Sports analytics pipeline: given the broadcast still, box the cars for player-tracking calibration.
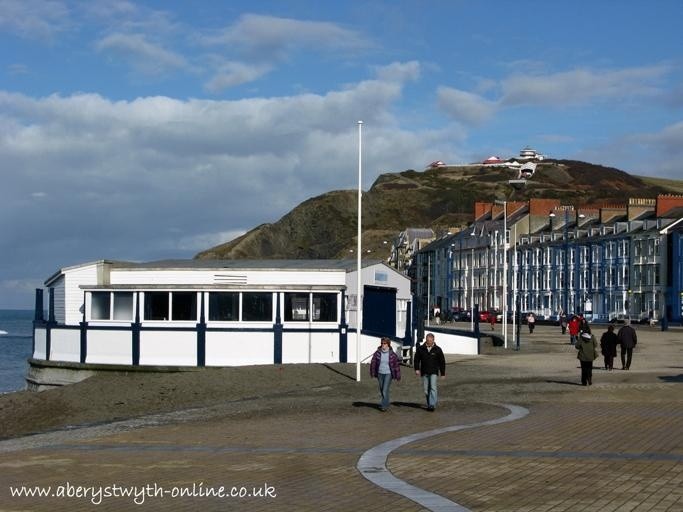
[438,309,537,324]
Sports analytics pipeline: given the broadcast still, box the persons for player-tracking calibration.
[423,309,457,327]
[414,333,446,412]
[490,314,496,330]
[370,336,401,412]
[527,313,536,334]
[649,308,656,328]
[559,310,588,346]
[574,326,598,386]
[600,326,618,371]
[617,319,637,370]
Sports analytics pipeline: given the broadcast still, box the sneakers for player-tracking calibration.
[605,367,629,371]
[582,379,592,386]
[424,404,435,411]
[379,405,389,411]
